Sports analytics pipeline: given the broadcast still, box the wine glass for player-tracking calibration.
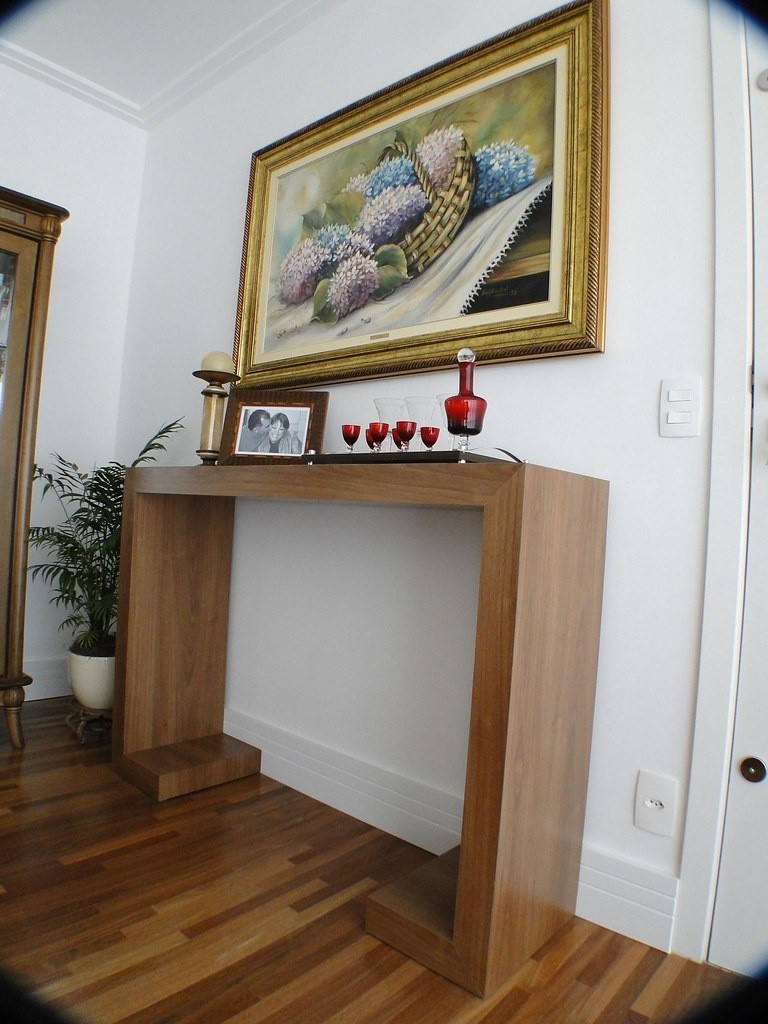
[341,421,440,453]
[373,397,405,452]
[404,396,443,451]
[436,393,458,451]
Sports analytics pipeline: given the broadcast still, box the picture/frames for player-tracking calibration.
[232,0,610,392]
[218,389,329,464]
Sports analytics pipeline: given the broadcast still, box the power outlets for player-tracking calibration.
[634,769,679,836]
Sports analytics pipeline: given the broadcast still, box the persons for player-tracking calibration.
[239,410,271,451]
[259,413,302,454]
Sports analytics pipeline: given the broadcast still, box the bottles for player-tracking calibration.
[444,347,487,451]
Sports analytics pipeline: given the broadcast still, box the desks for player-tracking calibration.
[107,461,609,999]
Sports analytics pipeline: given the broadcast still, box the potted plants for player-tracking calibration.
[26,419,185,745]
